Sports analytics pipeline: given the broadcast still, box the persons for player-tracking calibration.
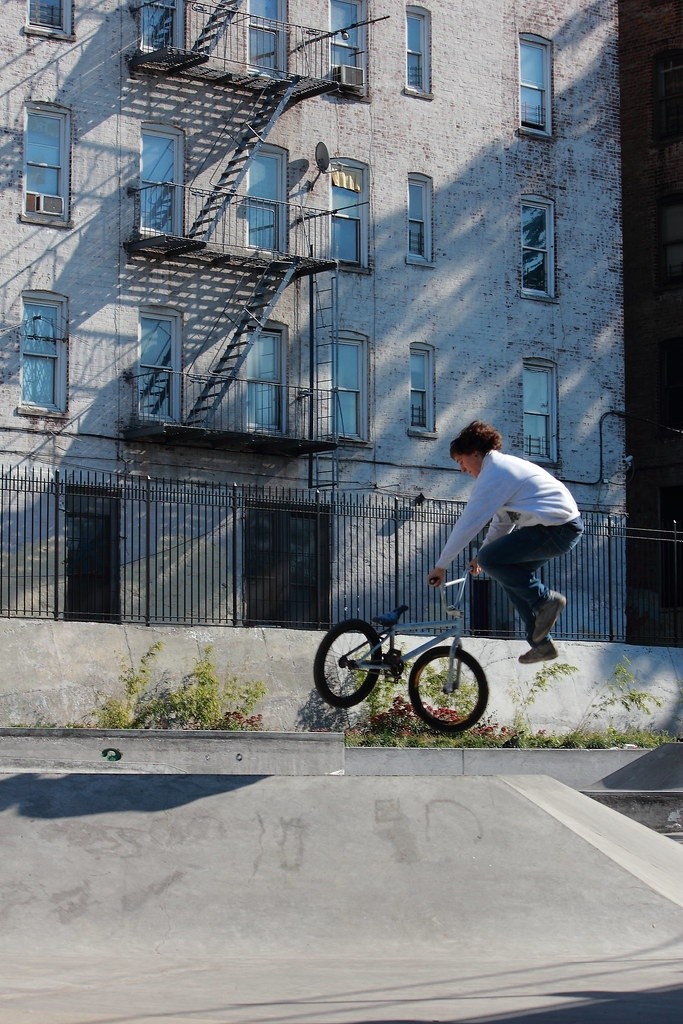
[424,420,584,662]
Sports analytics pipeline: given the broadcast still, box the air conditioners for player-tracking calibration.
[334,64,365,88]
[33,194,65,216]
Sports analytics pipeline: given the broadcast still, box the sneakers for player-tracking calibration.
[532,589,567,644]
[518,639,559,664]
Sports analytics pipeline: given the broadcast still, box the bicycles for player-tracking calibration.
[311,563,496,736]
